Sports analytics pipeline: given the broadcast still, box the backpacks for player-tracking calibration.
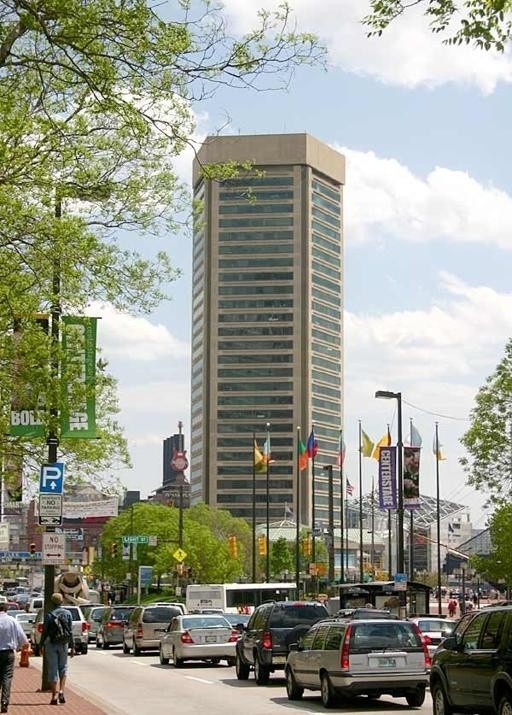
[49,610,73,645]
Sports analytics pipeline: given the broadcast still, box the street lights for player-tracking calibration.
[423,569,426,584]
[413,567,417,582]
[453,561,476,616]
[323,464,336,582]
[376,388,407,617]
[366,531,375,561]
[40,179,117,698]
[475,573,481,611]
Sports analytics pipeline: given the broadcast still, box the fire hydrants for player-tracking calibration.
[19,642,29,667]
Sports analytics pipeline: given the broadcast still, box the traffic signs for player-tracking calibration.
[41,532,65,566]
[121,536,148,544]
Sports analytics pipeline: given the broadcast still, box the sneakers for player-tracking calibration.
[50,699,57,705]
[59,692,65,703]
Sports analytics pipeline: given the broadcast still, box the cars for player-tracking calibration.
[405,617,458,673]
[0,574,44,636]
[158,613,239,669]
[221,613,251,632]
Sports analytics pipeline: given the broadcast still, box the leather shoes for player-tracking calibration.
[2,702,7,713]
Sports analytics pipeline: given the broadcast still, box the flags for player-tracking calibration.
[298,439,306,472]
[263,437,273,466]
[306,430,318,459]
[411,425,423,449]
[337,441,345,468]
[254,440,266,474]
[374,433,391,463]
[346,477,354,496]
[286,504,292,514]
[433,435,447,460]
[359,429,373,458]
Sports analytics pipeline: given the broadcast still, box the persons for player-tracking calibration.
[1,603,30,713]
[426,586,503,618]
[38,593,75,705]
[109,590,124,603]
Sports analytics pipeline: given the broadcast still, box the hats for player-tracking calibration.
[51,592,64,605]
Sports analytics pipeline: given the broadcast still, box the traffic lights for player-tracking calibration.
[259,536,266,558]
[227,536,237,560]
[302,537,310,558]
[110,542,117,560]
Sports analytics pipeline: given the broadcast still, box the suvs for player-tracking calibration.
[284,608,433,709]
[234,600,330,685]
[430,604,512,715]
[27,601,187,660]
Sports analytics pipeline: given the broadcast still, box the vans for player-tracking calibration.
[188,608,224,616]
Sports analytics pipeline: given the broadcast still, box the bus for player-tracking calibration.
[185,582,298,617]
[287,571,375,599]
